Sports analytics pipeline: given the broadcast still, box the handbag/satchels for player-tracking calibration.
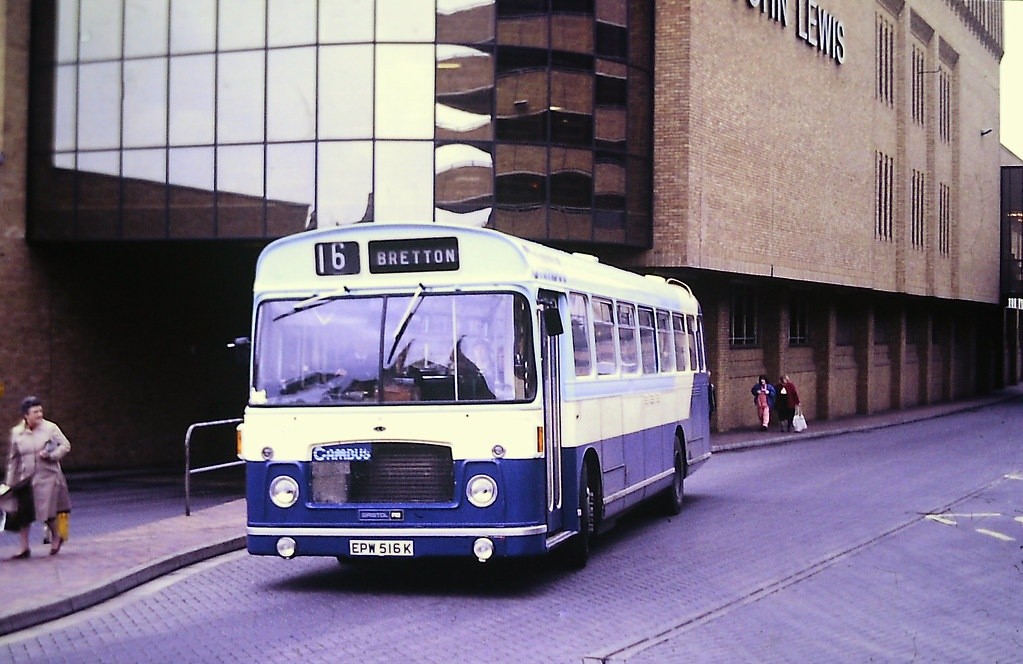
[793,404,808,433]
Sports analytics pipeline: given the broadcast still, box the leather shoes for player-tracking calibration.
[49,537,63,556]
[9,548,30,559]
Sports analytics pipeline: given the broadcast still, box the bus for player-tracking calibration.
[237,222,709,574]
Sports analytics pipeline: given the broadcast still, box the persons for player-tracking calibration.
[751,375,777,431]
[386,343,524,399]
[706,371,717,421]
[4,395,71,560]
[775,374,802,434]
